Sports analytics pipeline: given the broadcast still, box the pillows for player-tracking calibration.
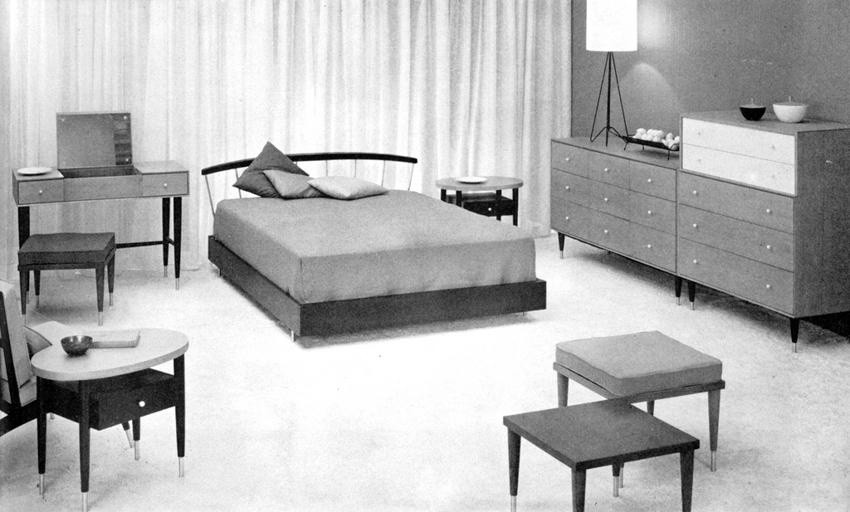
[233,141,309,199]
[23,325,53,356]
[262,170,324,199]
[307,175,389,199]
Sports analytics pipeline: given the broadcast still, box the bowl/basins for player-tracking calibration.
[61,335,92,356]
[772,101,809,124]
[740,105,766,120]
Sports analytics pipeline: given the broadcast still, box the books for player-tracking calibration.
[88,329,141,348]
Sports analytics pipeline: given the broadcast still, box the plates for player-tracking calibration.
[457,177,487,184]
[18,166,52,175]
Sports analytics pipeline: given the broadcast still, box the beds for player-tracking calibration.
[200,153,547,347]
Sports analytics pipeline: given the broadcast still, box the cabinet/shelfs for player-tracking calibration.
[12,112,190,279]
[551,137,681,304]
[677,104,848,344]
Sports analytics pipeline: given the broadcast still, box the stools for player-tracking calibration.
[503,398,700,512]
[15,233,116,327]
[553,331,726,487]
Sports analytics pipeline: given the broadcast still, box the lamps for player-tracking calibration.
[586,0,638,146]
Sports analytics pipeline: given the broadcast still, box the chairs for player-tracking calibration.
[0,280,82,438]
